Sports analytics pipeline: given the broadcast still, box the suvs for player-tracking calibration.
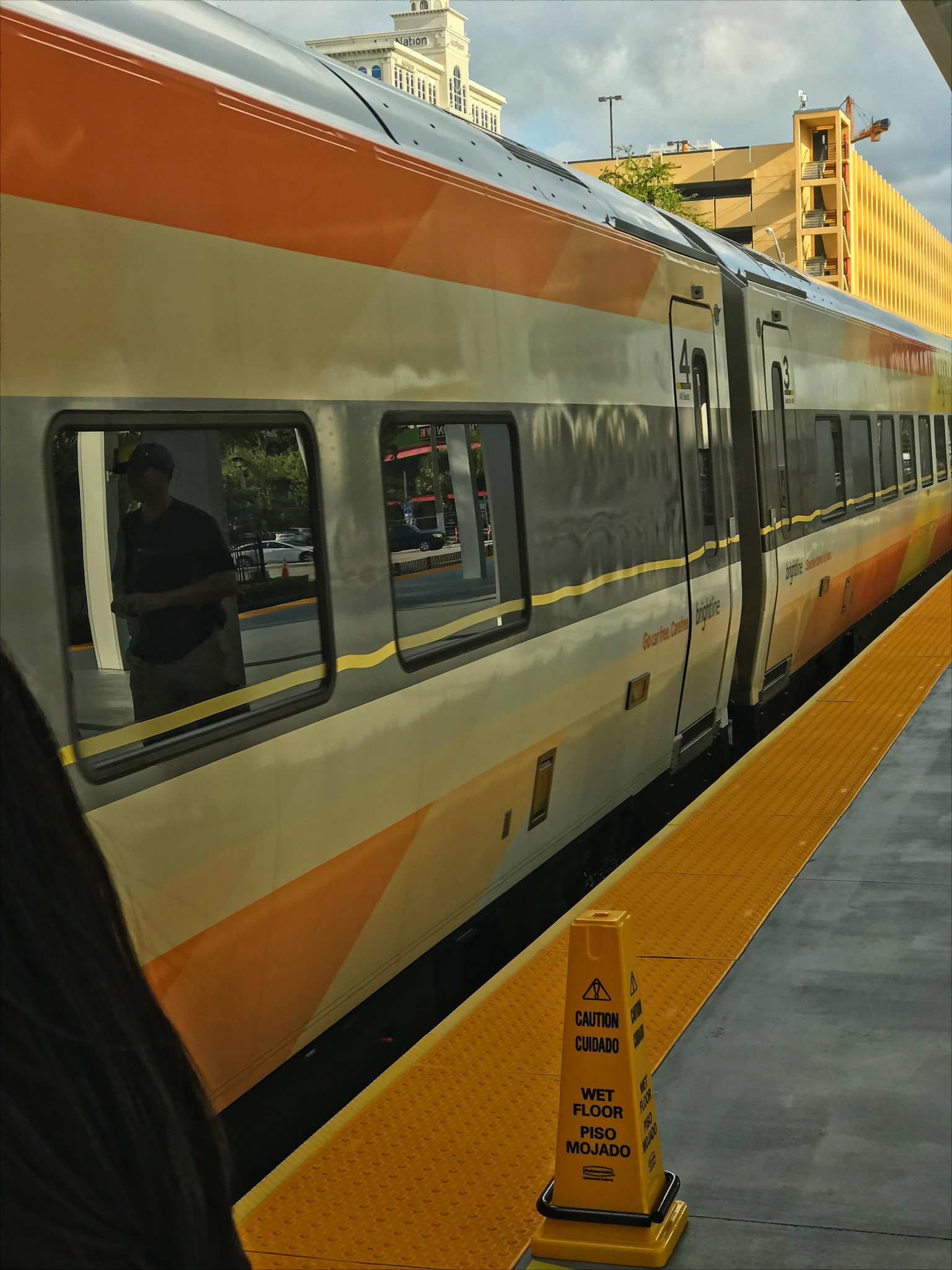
[388,524,444,552]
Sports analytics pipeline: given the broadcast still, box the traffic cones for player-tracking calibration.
[281,558,289,577]
[532,908,688,1268]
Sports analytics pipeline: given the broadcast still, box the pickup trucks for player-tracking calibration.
[275,527,313,546]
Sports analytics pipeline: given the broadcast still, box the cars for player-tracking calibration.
[230,541,313,567]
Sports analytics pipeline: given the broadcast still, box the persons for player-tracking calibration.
[109,442,247,748]
[0,646,247,1270]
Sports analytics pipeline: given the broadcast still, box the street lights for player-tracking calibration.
[598,95,623,157]
[667,141,689,152]
[765,227,784,263]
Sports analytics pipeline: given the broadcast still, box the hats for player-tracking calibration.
[114,441,174,474]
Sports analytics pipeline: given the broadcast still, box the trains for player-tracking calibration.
[1,2,951,1116]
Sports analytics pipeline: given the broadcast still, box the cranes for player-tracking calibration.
[845,96,892,149]
